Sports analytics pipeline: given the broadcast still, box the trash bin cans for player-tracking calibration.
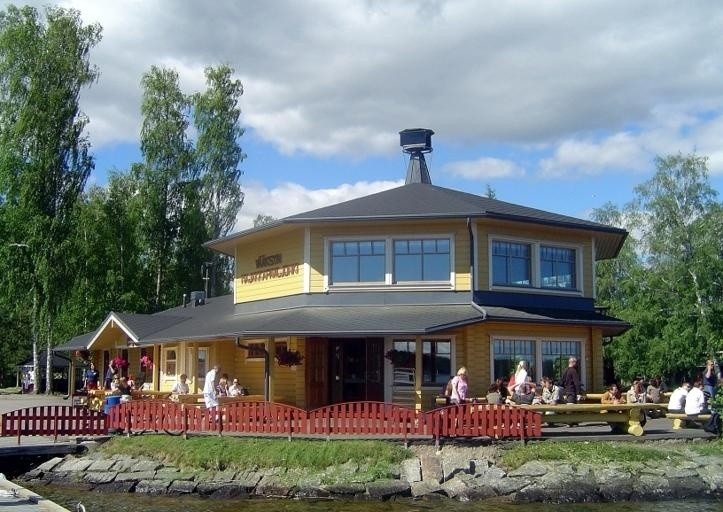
[104,396,121,413]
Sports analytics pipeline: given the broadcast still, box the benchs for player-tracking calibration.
[89,389,265,412]
[436,392,713,436]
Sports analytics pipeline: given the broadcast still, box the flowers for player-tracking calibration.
[275,350,303,368]
[140,355,153,370]
[113,355,128,368]
[386,350,415,366]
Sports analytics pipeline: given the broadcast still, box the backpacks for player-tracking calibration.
[703,413,721,434]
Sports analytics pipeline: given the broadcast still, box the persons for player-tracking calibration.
[442,354,717,428]
[80,359,245,423]
[27,367,34,383]
